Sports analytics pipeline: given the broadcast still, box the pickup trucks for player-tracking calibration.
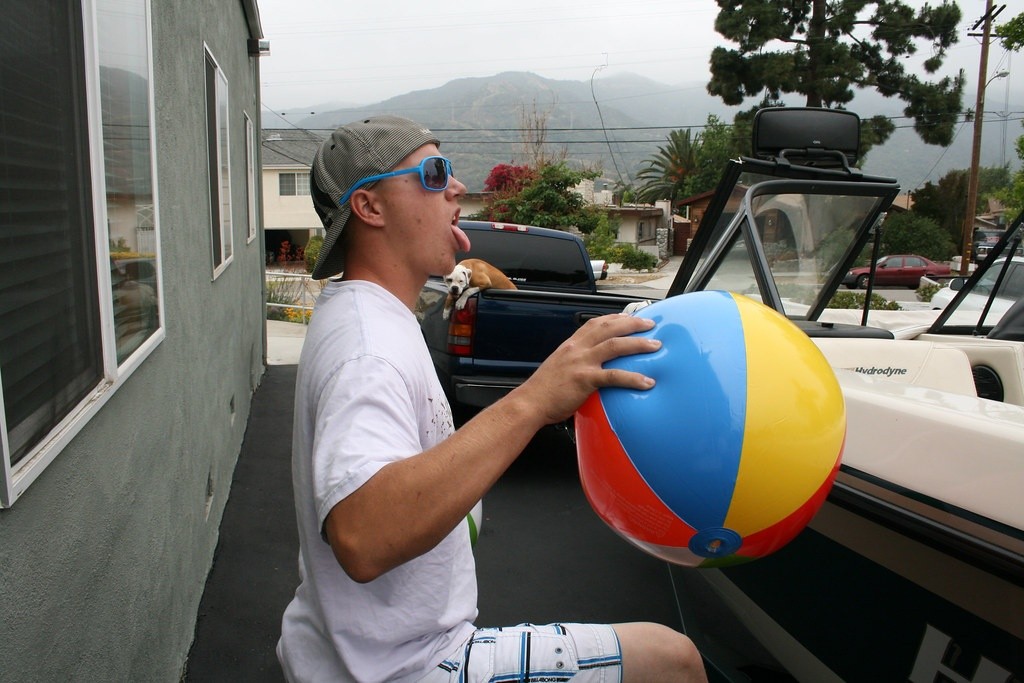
[111,260,158,305]
[415,222,667,450]
[590,259,607,280]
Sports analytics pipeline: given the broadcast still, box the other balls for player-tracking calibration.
[575,290,847,569]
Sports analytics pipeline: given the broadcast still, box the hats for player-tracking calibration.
[310,115,441,281]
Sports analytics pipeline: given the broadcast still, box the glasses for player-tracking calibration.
[340,156,454,205]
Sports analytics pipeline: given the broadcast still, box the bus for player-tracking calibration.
[975,228,1007,244]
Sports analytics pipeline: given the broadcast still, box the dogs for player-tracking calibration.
[442,258,518,320]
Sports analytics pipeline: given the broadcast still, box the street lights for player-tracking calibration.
[960,71,1011,282]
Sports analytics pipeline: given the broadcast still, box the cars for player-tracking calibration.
[840,254,952,288]
[931,257,1024,311]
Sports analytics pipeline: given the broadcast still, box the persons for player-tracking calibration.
[276,115,708,683]
